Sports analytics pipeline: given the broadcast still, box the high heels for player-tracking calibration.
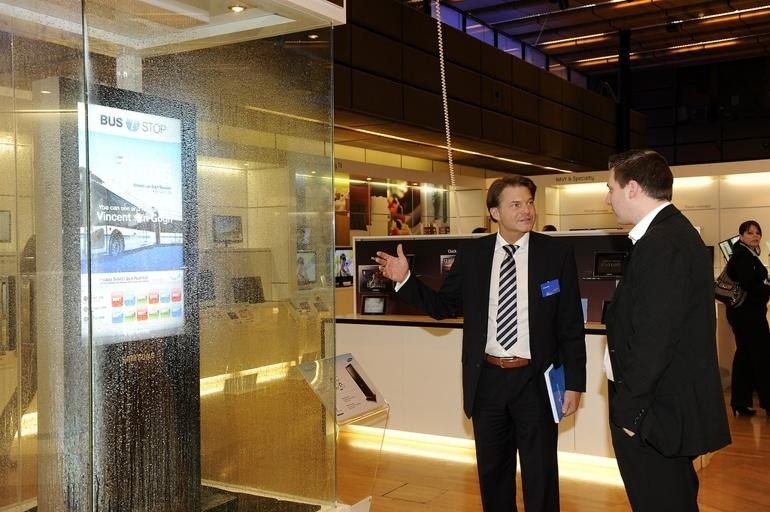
[731,403,756,416]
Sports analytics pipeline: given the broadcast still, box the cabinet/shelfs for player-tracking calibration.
[244,163,450,314]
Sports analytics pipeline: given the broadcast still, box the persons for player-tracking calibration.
[603,149,731,511]
[336,254,351,277]
[375,174,586,512]
[472,227,488,233]
[541,225,557,231]
[725,220,770,421]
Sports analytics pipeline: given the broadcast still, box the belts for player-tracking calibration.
[484,355,531,370]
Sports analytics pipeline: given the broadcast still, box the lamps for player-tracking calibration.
[228,4,247,14]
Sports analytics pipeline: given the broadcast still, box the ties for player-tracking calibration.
[496,245,519,351]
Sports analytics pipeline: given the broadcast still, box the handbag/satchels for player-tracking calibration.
[713,269,747,309]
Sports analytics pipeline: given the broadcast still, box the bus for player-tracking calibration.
[78,168,158,259]
[156,218,184,246]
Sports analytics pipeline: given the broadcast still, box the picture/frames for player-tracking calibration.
[0,276,16,351]
[718,234,761,264]
[0,210,11,243]
[297,251,317,284]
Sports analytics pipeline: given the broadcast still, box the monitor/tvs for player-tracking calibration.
[334,246,353,287]
[296,228,312,250]
[231,276,265,303]
[296,250,317,289]
[592,251,630,280]
[211,215,243,246]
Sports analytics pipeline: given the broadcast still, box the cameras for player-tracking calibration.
[358,265,393,295]
